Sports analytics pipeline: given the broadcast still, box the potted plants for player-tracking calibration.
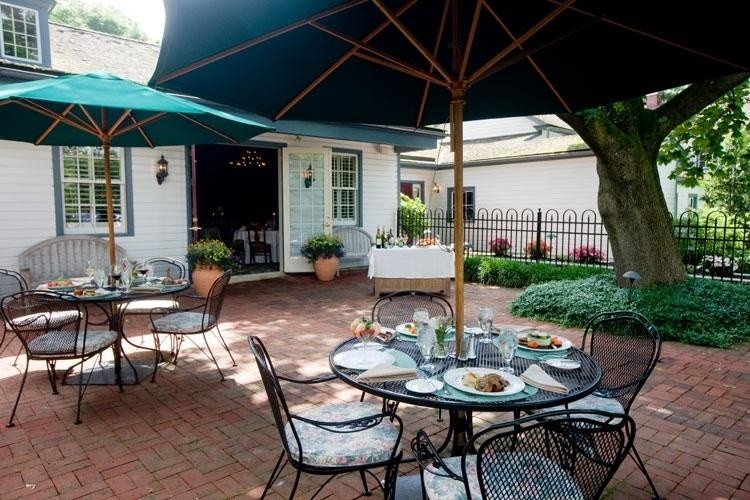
[301,234,344,281]
[185,238,234,295]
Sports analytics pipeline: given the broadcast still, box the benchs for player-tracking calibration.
[331,226,373,277]
[18,234,129,290]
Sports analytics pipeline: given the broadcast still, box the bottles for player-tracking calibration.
[458,333,477,361]
[376,224,393,248]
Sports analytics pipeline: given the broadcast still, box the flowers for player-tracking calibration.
[487,237,511,255]
[569,243,603,260]
[349,317,381,342]
[524,238,551,255]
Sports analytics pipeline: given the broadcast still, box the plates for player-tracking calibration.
[332,349,395,370]
[444,366,525,395]
[547,358,582,371]
[352,342,382,350]
[67,276,96,284]
[396,322,418,336]
[405,378,444,394]
[516,332,571,351]
[66,291,111,298]
[153,281,188,289]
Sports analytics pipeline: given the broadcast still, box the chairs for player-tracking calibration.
[409,408,636,500]
[371,290,454,422]
[247,334,403,500]
[518,311,664,499]
[247,223,272,267]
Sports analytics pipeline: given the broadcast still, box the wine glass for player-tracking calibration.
[498,329,518,374]
[418,327,437,371]
[94,270,105,289]
[479,305,493,344]
[413,309,429,346]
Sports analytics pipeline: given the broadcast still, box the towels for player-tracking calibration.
[520,363,569,395]
[354,362,417,384]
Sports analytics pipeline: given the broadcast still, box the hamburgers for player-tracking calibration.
[85,289,96,296]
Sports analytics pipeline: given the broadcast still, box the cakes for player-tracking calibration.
[526,330,552,346]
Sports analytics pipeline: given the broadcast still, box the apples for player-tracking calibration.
[74,288,83,296]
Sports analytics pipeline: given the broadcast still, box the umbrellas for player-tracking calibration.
[147,0,750,420]
[0,73,276,314]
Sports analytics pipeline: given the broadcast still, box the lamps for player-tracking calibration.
[156,153,168,185]
[304,163,316,187]
[433,181,440,194]
